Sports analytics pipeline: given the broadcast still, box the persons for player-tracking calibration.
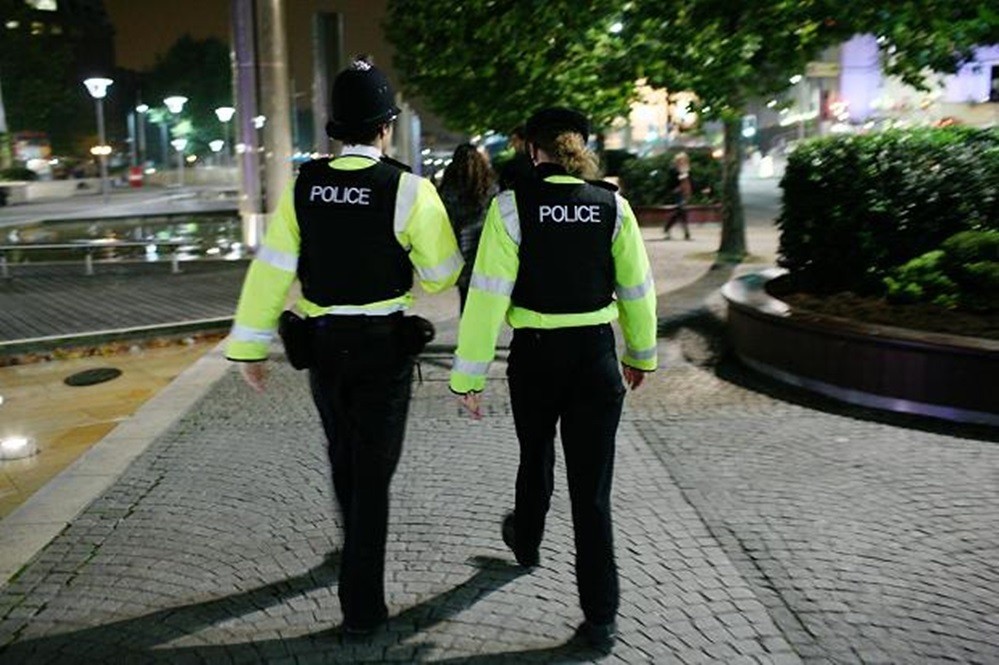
[661,151,711,243]
[437,143,498,316]
[449,100,658,652]
[491,124,537,190]
[219,52,466,639]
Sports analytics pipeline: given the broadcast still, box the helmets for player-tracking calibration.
[332,58,401,126]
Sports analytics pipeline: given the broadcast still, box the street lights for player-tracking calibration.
[210,105,265,175]
[85,77,114,193]
[162,95,189,192]
[134,103,150,163]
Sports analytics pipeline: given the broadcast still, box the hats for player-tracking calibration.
[527,107,589,143]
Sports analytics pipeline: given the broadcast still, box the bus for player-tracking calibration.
[1,129,50,170]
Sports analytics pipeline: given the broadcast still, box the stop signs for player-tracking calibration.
[127,165,145,187]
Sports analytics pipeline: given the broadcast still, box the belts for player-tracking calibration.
[314,319,399,329]
[516,327,611,336]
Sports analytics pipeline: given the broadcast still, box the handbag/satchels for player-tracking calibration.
[277,313,310,370]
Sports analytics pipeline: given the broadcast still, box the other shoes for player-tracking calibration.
[578,620,617,648]
[504,514,540,566]
[345,606,389,640]
